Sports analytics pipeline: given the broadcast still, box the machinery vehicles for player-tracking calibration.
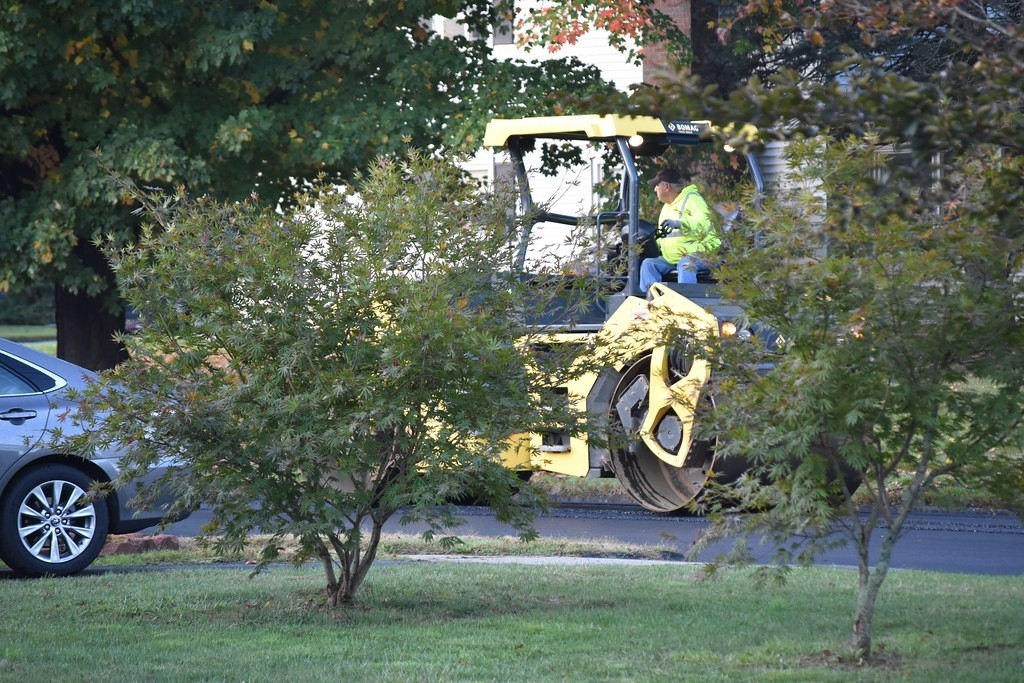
[299,113,875,515]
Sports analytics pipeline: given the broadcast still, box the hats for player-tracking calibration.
[646,167,684,186]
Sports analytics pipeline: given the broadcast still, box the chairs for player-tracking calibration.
[665,201,739,281]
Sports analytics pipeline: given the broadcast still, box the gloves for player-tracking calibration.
[660,218,682,235]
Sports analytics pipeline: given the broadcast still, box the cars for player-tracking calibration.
[0,335,212,580]
[124,279,205,337]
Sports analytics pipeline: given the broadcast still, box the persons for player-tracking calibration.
[640,167,726,293]
